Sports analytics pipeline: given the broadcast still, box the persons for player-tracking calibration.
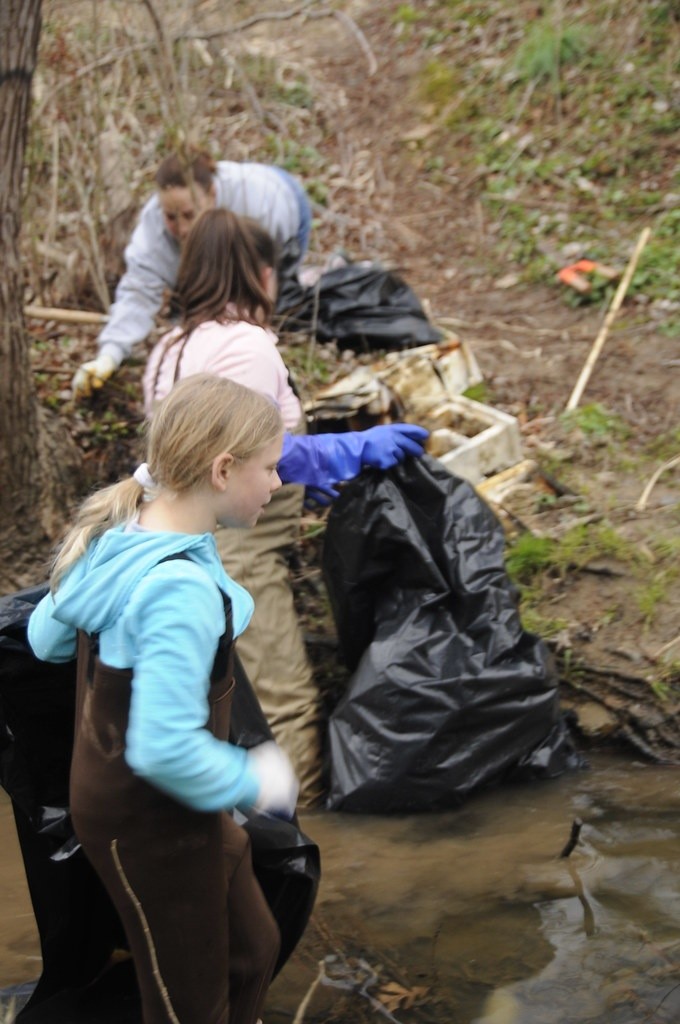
[143,208,429,814]
[27,370,301,1024]
[74,139,311,407]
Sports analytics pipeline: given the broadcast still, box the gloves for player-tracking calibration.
[69,352,127,408]
[247,740,300,821]
[276,423,429,509]
